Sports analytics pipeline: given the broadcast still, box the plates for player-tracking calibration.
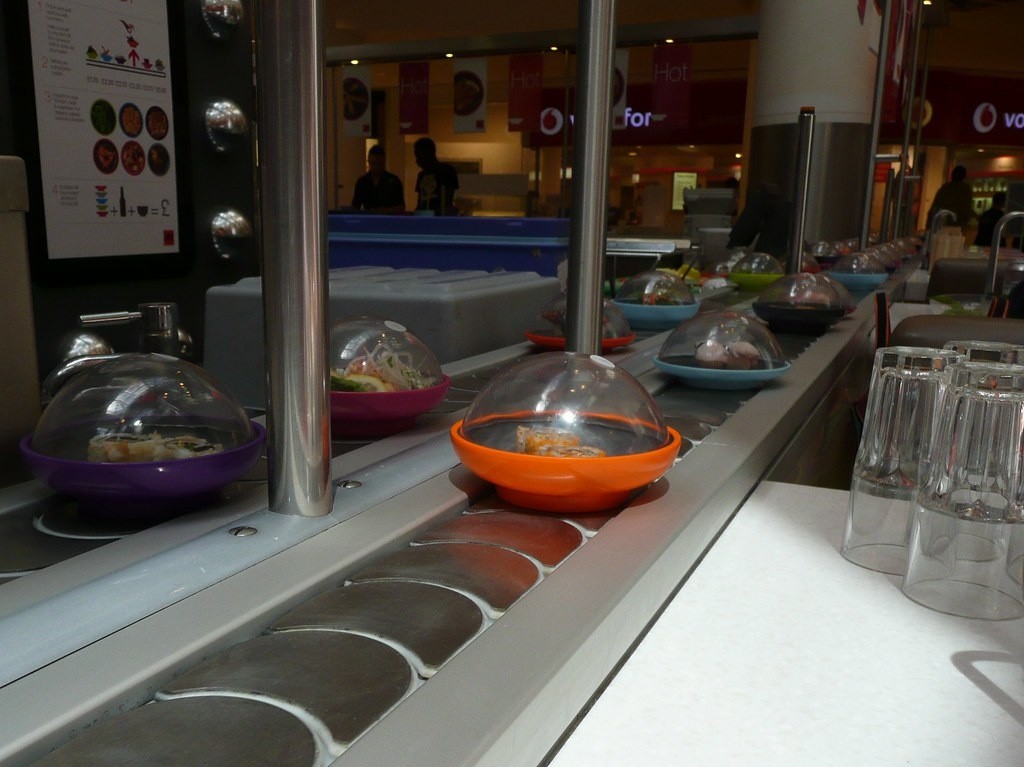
[119,103,143,138]
[92,138,119,175]
[89,99,117,136]
[120,140,145,176]
[344,77,368,121]
[145,105,169,141]
[613,66,624,109]
[454,70,483,115]
[147,143,170,177]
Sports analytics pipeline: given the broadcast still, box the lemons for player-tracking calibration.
[343,375,387,392]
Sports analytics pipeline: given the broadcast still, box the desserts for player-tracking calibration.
[693,339,760,369]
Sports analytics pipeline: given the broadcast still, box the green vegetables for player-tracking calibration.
[328,375,366,392]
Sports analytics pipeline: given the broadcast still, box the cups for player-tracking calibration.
[843,341,1023,621]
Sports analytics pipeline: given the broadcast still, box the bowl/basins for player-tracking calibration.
[813,255,844,265]
[19,413,267,506]
[330,374,450,436]
[752,301,845,332]
[822,269,888,290]
[930,293,995,318]
[652,353,792,391]
[609,298,701,329]
[841,241,922,274]
[524,327,636,354]
[451,413,681,514]
[728,272,785,289]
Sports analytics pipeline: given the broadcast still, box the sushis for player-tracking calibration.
[87,432,225,463]
[516,424,607,458]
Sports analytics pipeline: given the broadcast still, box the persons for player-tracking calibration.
[974,192,1008,246]
[727,162,790,252]
[413,136,459,217]
[350,144,406,213]
[924,164,972,231]
